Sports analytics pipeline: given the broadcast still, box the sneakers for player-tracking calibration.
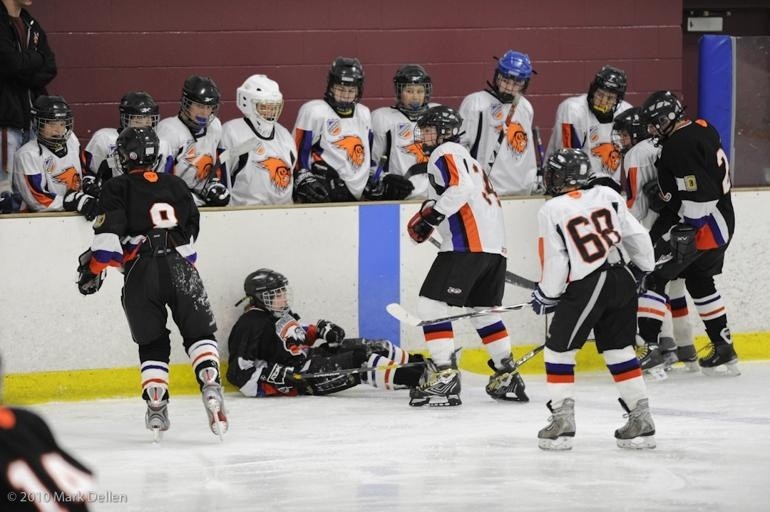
[410,346,461,397]
[195,360,229,435]
[486,352,524,394]
[634,329,737,376]
[143,387,170,432]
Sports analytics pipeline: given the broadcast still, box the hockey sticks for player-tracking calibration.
[387,303,533,326]
[208,138,260,183]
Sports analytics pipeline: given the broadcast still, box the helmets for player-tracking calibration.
[586,65,628,117]
[544,148,591,196]
[413,105,462,155]
[110,91,163,173]
[611,90,689,157]
[493,49,532,102]
[30,95,74,149]
[393,63,432,117]
[325,55,365,111]
[180,74,221,129]
[244,268,293,318]
[236,73,284,138]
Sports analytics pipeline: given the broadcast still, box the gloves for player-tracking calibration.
[644,180,665,211]
[382,174,414,200]
[670,227,697,263]
[407,198,445,244]
[531,282,561,315]
[205,185,230,207]
[78,247,107,295]
[294,171,329,203]
[63,176,103,221]
[317,318,345,343]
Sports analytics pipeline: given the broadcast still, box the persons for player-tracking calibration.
[82,90,175,191]
[216,72,299,208]
[0,0,58,215]
[226,268,437,397]
[633,90,737,371]
[454,49,548,198]
[406,104,527,399]
[76,124,230,437]
[0,405,89,511]
[540,65,636,200]
[288,55,374,205]
[612,106,699,367]
[12,93,102,221]
[526,146,656,440]
[364,63,445,203]
[155,72,230,208]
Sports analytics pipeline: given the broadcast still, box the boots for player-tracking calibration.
[616,398,655,439]
[538,399,575,439]
